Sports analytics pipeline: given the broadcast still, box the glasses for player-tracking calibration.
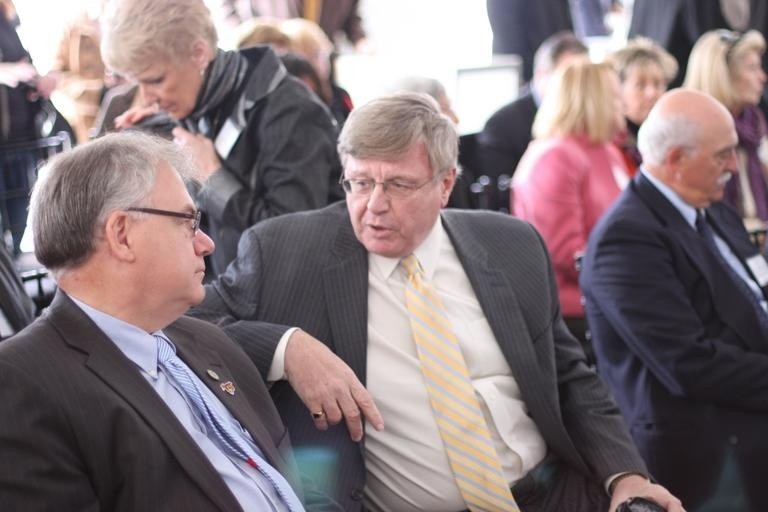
[339,162,442,197]
[125,207,203,234]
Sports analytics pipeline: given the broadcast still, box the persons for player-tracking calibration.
[98,1,342,299]
[231,23,320,114]
[0,0,57,222]
[510,61,631,365]
[471,31,589,231]
[604,0,632,42]
[377,72,469,212]
[581,89,768,512]
[181,92,690,512]
[0,131,308,512]
[607,38,679,182]
[272,18,355,126]
[683,26,768,259]
[0,225,40,341]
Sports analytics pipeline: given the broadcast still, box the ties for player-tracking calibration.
[153,334,307,511]
[697,211,768,338]
[403,254,521,512]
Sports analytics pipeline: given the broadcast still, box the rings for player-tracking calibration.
[308,410,324,422]
